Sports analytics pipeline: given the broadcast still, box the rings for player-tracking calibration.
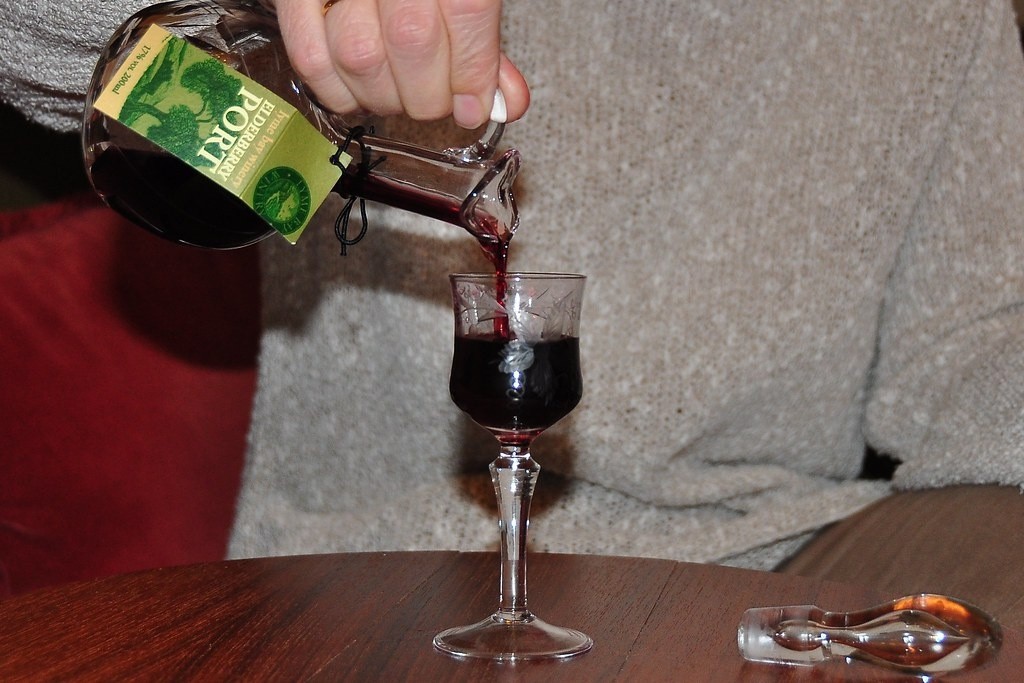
[319,0,342,17]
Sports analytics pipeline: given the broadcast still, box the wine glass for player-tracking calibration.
[432,272,594,662]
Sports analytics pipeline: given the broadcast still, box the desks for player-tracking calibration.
[0,550,1024,683]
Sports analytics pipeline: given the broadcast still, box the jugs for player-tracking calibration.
[82,0,521,250]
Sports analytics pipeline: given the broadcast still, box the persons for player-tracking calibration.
[0,0,1024,572]
